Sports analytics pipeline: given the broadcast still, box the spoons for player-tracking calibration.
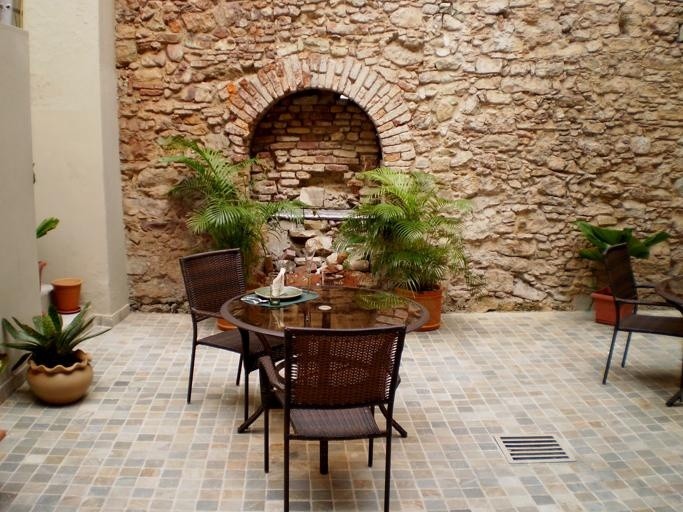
[251,298,269,303]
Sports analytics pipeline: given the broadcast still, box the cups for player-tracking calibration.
[271,273,280,305]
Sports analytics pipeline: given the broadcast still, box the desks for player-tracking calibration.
[221,286,430,474]
[656,273,683,407]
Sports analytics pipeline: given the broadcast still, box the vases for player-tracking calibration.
[51,278,82,314]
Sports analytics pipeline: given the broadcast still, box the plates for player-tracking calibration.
[255,286,303,299]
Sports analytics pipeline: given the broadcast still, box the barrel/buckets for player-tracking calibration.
[395,282,443,333]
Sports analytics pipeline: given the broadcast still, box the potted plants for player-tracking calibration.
[157,139,278,330]
[331,163,483,333]
[573,221,671,328]
[35,216,59,294]
[0,302,111,407]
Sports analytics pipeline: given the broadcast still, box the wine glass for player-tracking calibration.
[302,247,316,291]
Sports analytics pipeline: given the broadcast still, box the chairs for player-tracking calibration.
[602,243,683,384]
[177,247,297,403]
[258,328,407,512]
